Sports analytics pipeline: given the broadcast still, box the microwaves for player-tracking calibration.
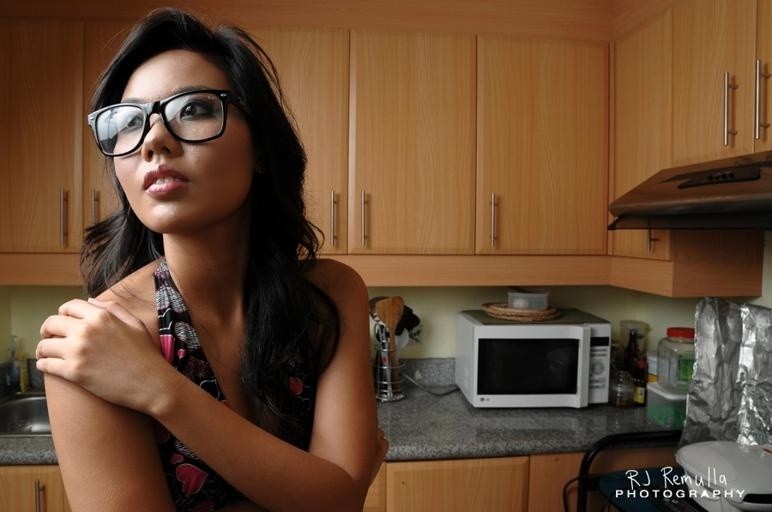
[454,307,611,409]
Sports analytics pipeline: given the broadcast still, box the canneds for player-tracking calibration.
[659,325,695,393]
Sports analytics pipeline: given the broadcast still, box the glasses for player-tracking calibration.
[87,89,248,157]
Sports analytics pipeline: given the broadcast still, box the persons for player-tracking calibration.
[36,7,388,512]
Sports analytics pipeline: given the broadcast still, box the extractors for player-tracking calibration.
[606,149,772,230]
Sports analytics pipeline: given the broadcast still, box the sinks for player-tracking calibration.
[0,398,52,436]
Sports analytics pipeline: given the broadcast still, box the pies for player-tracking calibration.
[482,302,560,322]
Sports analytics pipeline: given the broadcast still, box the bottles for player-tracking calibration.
[611,327,695,410]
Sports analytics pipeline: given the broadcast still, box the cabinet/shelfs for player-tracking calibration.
[389,451,677,511]
[0,463,388,511]
[0,16,143,253]
[607,0,772,262]
[247,28,476,256]
[578,429,683,512]
[476,35,615,257]
